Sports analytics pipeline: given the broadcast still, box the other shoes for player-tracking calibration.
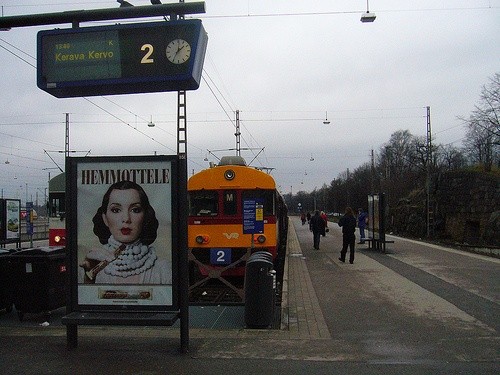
[349,260,353,263]
[339,258,345,263]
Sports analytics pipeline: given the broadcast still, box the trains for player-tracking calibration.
[182,110,290,307]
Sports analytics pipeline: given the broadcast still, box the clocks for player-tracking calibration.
[166,38,191,64]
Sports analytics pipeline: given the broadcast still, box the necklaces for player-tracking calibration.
[102,235,159,277]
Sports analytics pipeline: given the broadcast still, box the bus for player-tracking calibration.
[47,190,66,246]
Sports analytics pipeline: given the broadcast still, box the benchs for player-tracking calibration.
[385,240,394,243]
[362,237,372,241]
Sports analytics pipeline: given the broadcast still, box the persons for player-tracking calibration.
[79,179,172,285]
[299,210,328,250]
[338,207,355,265]
[358,208,367,244]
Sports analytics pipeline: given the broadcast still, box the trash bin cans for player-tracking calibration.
[0,245,67,322]
[243,250,276,329]
